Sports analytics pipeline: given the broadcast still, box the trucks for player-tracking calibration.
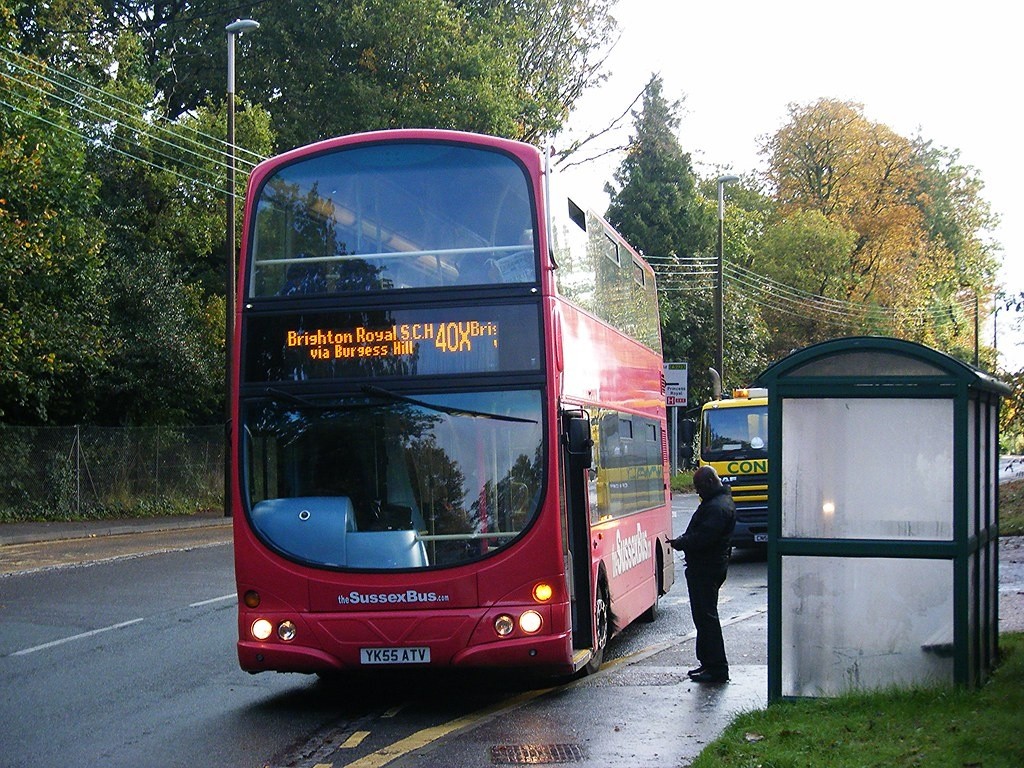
[689,365,770,561]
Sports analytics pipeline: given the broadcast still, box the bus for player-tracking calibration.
[229,129,674,685]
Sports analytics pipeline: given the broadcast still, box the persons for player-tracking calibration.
[663,463,738,685]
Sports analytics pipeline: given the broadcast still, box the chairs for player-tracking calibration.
[338,250,382,292]
[277,252,327,295]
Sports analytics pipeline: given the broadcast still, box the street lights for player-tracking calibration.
[716,174,741,391]
[222,18,261,519]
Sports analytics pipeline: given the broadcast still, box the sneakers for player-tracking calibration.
[688,667,729,682]
